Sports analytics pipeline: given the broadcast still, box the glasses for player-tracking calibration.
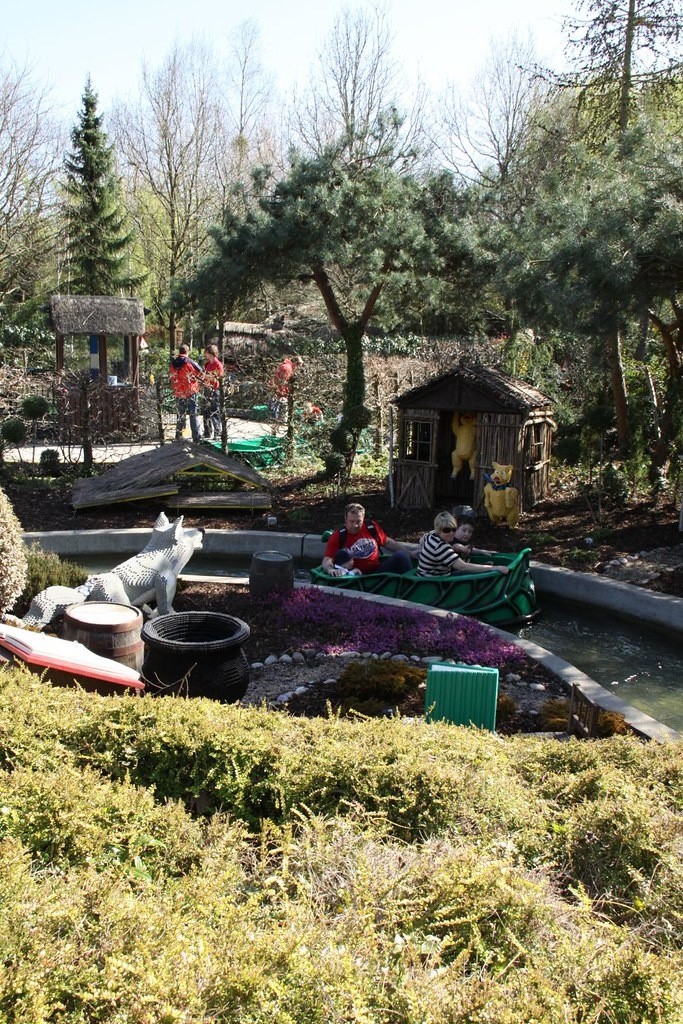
[441,527,457,534]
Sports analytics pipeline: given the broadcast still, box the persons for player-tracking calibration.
[301,399,323,426]
[169,345,224,443]
[267,356,304,436]
[333,548,362,576]
[415,506,509,578]
[322,503,421,577]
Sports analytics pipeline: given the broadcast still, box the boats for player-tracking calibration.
[252,402,330,422]
[210,424,318,469]
[163,389,207,415]
[311,530,542,631]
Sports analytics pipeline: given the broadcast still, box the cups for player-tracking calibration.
[109,376,117,386]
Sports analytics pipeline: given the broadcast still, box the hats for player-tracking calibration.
[332,548,355,565]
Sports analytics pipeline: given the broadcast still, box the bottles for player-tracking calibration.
[149,372,155,385]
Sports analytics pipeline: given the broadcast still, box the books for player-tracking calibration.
[0,623,145,689]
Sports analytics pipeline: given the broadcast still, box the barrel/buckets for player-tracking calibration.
[64,601,145,673]
[249,550,294,595]
[251,519,297,533]
